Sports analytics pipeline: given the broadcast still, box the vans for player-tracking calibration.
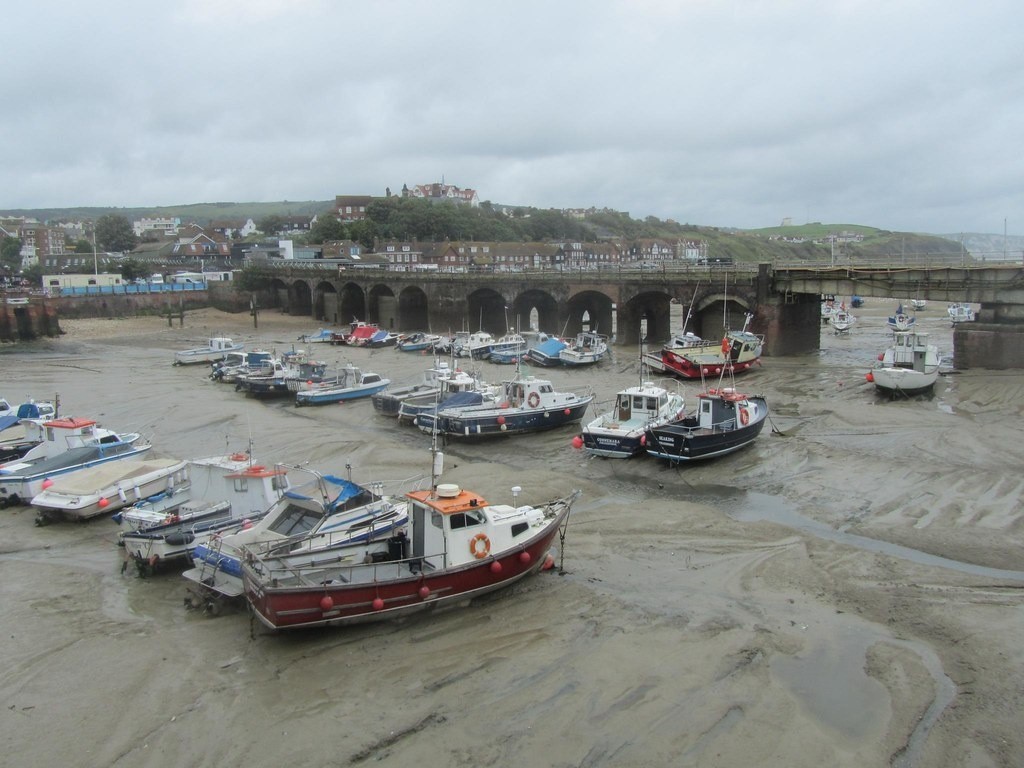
[151,273,164,285]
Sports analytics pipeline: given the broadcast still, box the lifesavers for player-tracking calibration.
[899,316,905,322]
[471,534,491,558]
[228,454,251,461]
[507,385,522,406]
[253,348,261,352]
[347,336,356,344]
[721,392,748,401]
[246,466,267,473]
[740,410,750,426]
[527,392,540,407]
[708,388,724,394]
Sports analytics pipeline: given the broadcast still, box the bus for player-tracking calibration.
[698,257,732,265]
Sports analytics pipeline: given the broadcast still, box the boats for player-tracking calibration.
[866,332,943,400]
[947,303,977,323]
[172,335,772,466]
[0,392,582,637]
[298,272,766,379]
[887,299,927,332]
[821,299,857,332]
